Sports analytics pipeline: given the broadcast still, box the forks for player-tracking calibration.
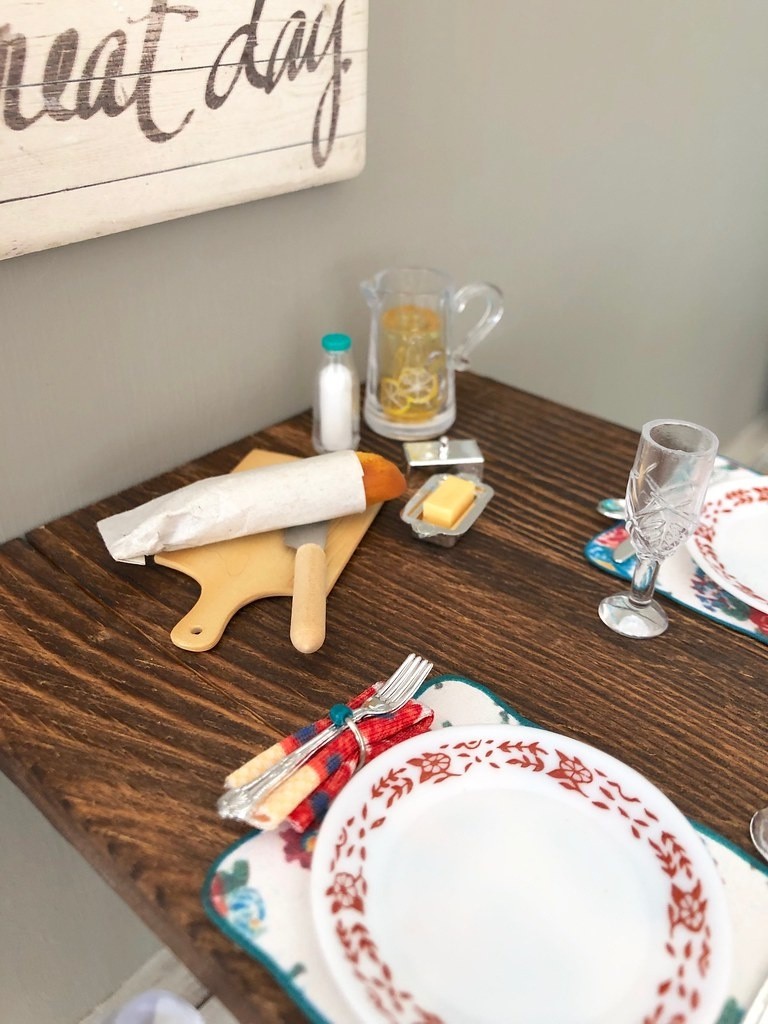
[214,651,434,822]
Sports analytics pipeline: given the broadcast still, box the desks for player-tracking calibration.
[0,369,768,1024]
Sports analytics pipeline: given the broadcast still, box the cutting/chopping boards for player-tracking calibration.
[154,445,387,652]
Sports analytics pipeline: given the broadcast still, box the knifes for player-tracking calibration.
[612,538,636,564]
[283,519,329,654]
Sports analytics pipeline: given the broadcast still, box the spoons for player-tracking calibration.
[749,806,768,862]
[596,464,740,520]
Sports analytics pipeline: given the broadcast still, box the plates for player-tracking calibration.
[685,475,768,615]
[306,723,732,1024]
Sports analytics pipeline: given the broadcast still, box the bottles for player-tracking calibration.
[310,333,361,455]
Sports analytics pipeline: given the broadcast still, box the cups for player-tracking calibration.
[359,266,503,442]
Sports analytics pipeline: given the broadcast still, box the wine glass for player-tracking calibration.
[596,419,720,639]
